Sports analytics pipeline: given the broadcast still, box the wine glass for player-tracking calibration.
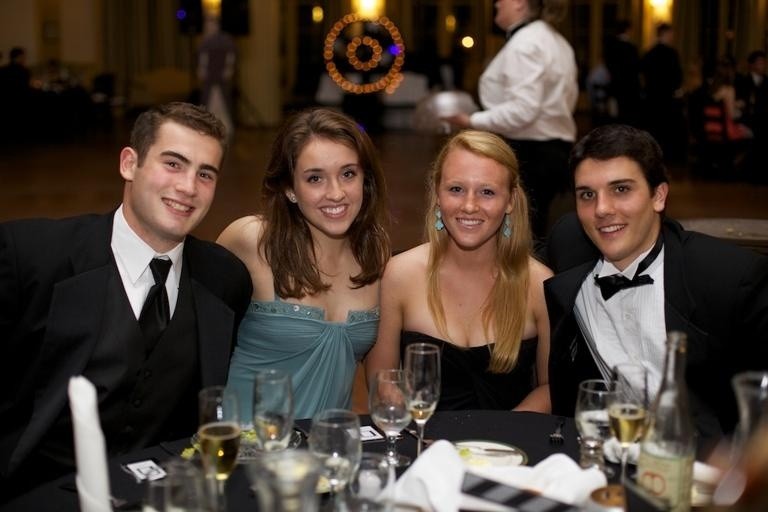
[368,368,414,466]
[198,385,243,511]
[404,344,440,457]
[574,365,650,509]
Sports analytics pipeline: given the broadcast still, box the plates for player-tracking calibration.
[237,424,303,465]
[451,437,529,473]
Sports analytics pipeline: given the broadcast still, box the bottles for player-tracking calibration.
[733,374,766,471]
[640,322,696,512]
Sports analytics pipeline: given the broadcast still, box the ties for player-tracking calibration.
[137,259,173,342]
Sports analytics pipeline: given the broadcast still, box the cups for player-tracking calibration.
[146,463,204,512]
[251,368,294,454]
[349,451,397,511]
[309,409,362,512]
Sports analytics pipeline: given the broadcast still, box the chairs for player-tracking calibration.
[694,97,756,183]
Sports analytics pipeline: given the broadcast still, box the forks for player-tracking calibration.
[549,416,566,444]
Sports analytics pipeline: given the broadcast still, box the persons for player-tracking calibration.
[1,45,73,139]
[1,103,253,495]
[542,124,768,433]
[362,129,555,415]
[587,16,767,179]
[444,0,579,251]
[212,106,382,424]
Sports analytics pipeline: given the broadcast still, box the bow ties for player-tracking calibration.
[592,270,655,301]
